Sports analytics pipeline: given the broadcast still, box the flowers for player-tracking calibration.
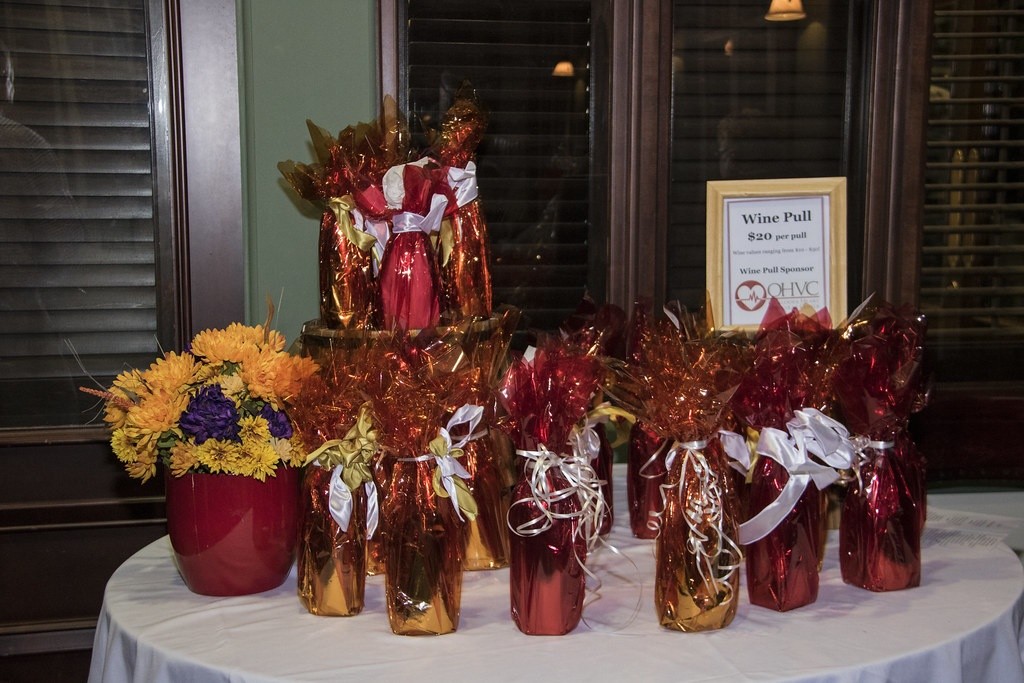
[64,284,321,487]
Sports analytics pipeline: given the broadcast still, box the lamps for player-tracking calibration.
[553,60,573,76]
[765,0,806,21]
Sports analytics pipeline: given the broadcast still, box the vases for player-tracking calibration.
[163,469,304,597]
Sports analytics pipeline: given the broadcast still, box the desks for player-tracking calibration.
[87,463,1024,683]
